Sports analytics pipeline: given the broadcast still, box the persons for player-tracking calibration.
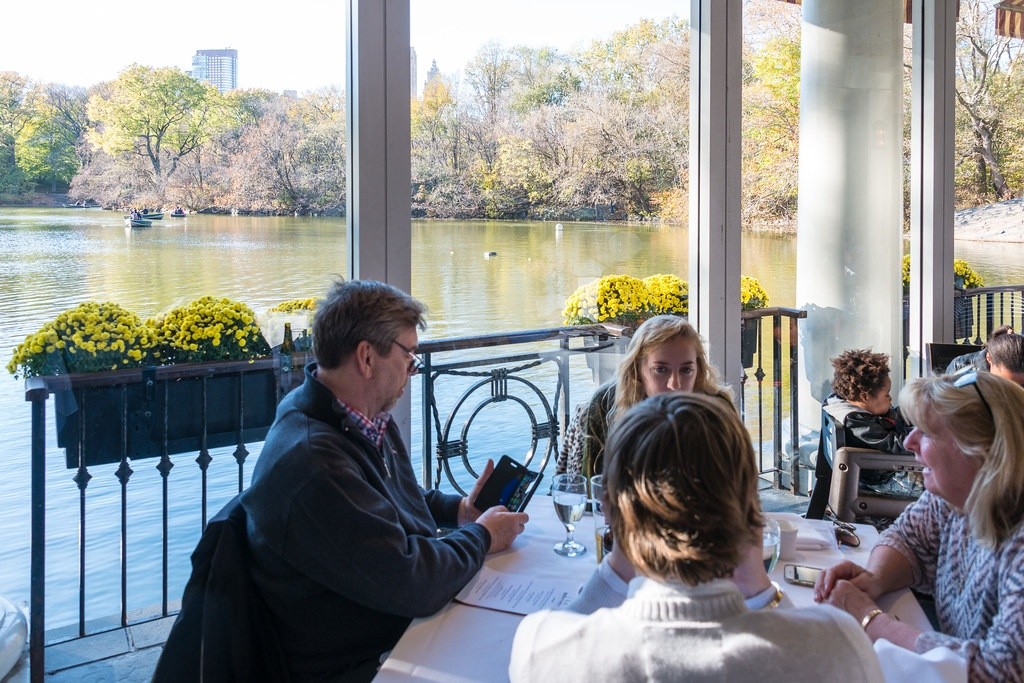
[822,347,913,456]
[130,207,142,220]
[509,392,885,682]
[813,368,1024,683]
[241,280,528,683]
[945,325,1024,385]
[547,315,733,496]
[143,206,148,214]
[175,207,182,213]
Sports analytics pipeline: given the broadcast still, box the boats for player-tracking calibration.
[123,219,152,227]
[66,203,91,208]
[170,206,186,217]
[92,201,122,210]
[124,213,164,220]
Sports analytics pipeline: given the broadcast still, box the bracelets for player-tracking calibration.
[765,591,783,610]
[862,610,882,626]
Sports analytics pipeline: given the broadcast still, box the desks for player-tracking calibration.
[371,495,934,683]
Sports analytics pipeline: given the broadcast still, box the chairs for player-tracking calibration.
[926,341,985,377]
[804,401,923,523]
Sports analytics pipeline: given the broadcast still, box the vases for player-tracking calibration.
[903,295,973,345]
[55,370,305,470]
[584,318,757,385]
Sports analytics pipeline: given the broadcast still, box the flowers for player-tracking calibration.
[902,254,984,295]
[561,273,770,325]
[5,296,321,380]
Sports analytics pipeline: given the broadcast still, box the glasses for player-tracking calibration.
[391,339,423,373]
[954,364,995,425]
[833,519,860,550]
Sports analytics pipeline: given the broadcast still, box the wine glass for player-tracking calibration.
[762,518,782,591]
[552,474,588,556]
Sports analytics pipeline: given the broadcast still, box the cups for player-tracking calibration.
[775,519,798,560]
[603,525,614,557]
[590,474,609,563]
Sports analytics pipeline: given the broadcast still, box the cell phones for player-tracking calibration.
[506,471,543,513]
[784,564,823,586]
